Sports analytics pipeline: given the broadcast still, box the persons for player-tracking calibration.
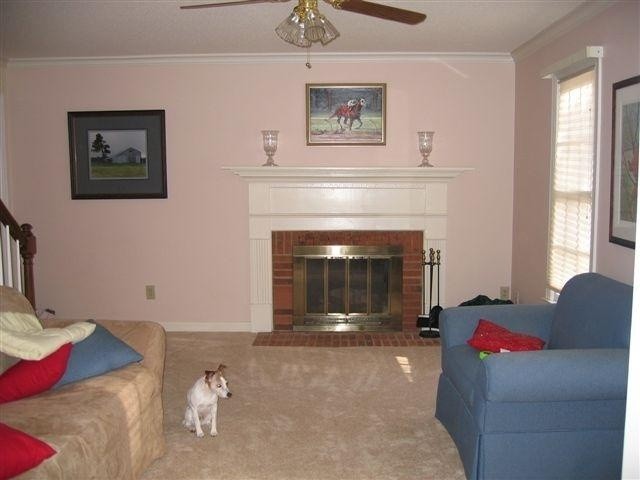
[347,99,356,112]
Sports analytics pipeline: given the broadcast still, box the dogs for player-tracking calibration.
[182,364,232,438]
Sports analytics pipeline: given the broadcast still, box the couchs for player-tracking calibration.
[0,281,167,480]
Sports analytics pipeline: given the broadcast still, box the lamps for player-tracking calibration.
[273,1,341,50]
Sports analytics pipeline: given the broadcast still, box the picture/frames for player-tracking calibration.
[67,110,167,201]
[305,83,387,147]
[608,76,639,251]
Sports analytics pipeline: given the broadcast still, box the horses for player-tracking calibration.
[328,96,367,136]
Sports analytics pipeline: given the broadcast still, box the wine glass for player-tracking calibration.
[418,132,434,168]
[261,130,280,167]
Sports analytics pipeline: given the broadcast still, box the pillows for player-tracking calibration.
[1,321,144,480]
[466,318,546,352]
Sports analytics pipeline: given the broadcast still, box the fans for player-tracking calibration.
[180,1,428,25]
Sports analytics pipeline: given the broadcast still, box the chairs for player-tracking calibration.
[434,273,633,479]
